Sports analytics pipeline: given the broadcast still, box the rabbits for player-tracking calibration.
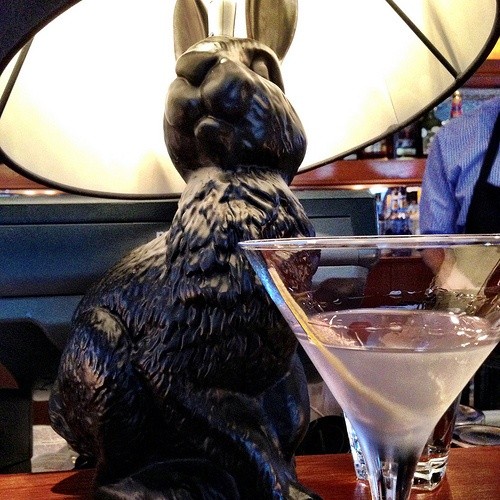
[48,1,322,500]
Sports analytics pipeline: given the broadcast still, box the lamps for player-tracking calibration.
[0,0,500,500]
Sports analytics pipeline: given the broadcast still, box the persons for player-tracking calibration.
[418,91,500,410]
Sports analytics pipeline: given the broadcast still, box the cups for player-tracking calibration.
[344,391,462,491]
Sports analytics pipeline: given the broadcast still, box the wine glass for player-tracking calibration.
[238,234,500,500]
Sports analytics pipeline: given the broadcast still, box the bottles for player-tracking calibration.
[450,90,462,118]
[418,109,442,158]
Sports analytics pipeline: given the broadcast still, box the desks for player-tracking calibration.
[0,445,500,500]
